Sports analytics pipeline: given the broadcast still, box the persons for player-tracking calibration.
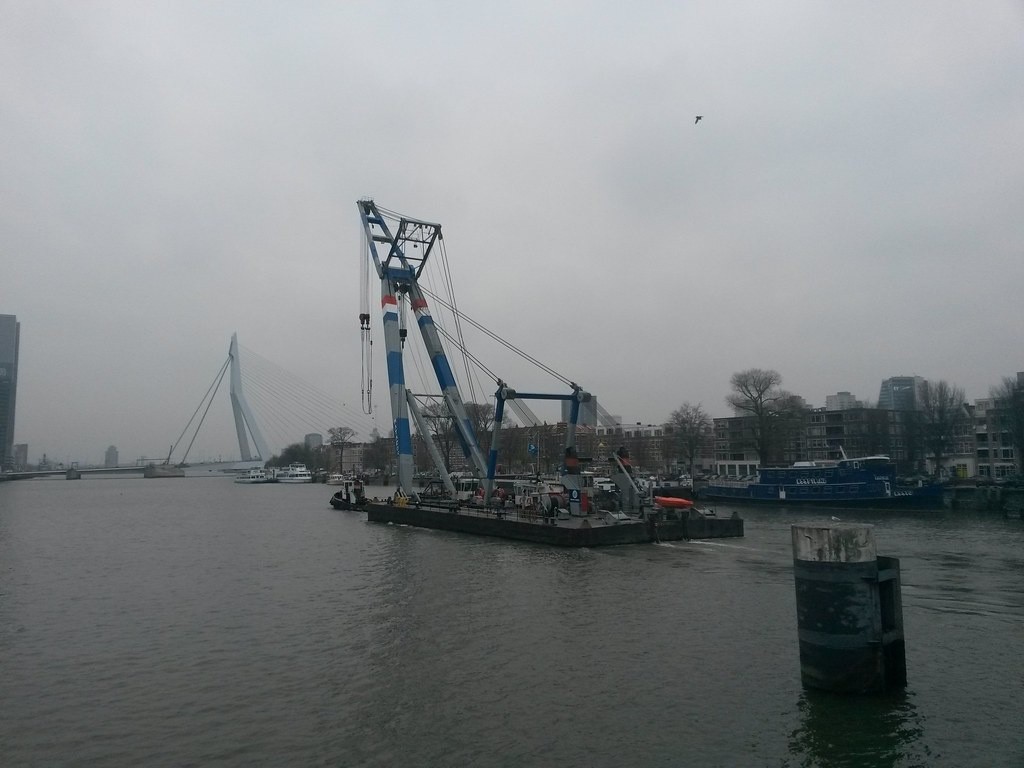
[639,500,644,518]
[589,499,599,513]
[476,488,484,497]
[508,490,515,501]
[498,488,505,498]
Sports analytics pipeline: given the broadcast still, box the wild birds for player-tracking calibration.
[694,115,704,124]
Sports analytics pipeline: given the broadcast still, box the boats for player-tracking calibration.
[329,463,373,512]
[655,496,694,509]
[706,445,944,509]
[234,462,345,485]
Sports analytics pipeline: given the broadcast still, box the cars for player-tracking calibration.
[649,472,757,481]
[895,475,960,487]
[974,475,1019,488]
